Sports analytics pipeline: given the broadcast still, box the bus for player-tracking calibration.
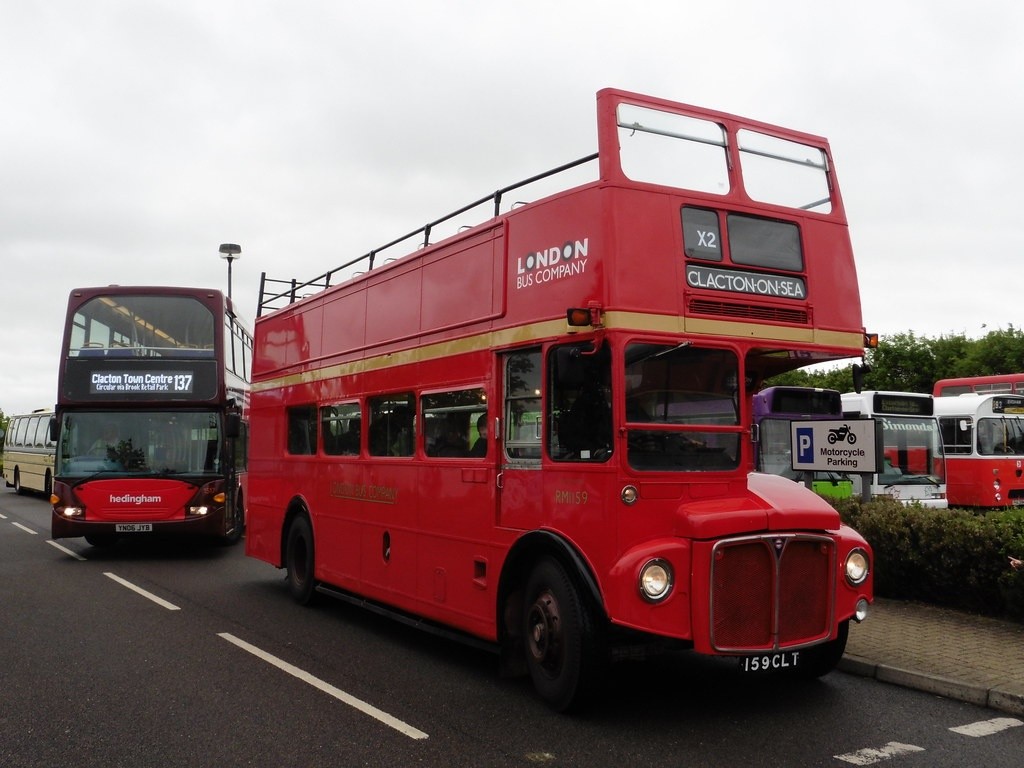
[3,409,58,495]
[652,386,853,499]
[932,373,1024,396]
[244,90,885,709]
[50,284,253,548]
[932,392,1024,509]
[843,391,949,509]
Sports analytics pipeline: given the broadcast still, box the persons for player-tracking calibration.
[89,423,122,457]
[472,413,487,458]
[566,362,681,457]
[318,418,467,457]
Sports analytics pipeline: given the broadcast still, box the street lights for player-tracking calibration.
[218,243,244,300]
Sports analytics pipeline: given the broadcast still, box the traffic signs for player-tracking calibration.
[788,418,878,473]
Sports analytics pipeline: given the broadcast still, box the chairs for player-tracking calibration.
[300,200,530,300]
[78,341,214,358]
